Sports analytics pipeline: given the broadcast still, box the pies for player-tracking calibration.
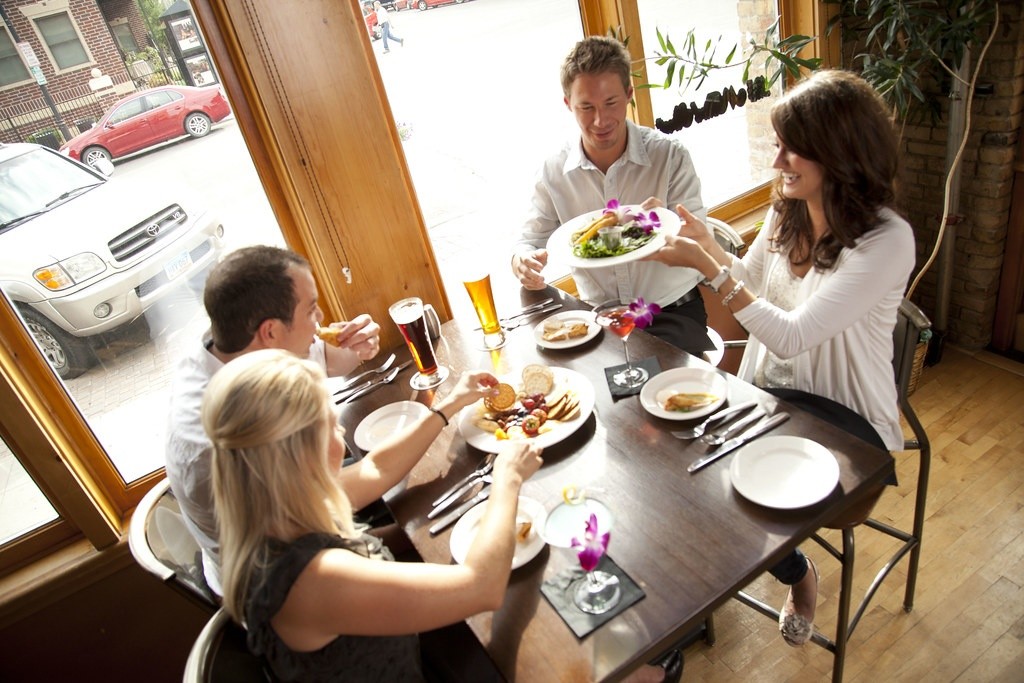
[655,390,719,411]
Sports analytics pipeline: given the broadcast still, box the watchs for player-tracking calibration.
[706,266,731,294]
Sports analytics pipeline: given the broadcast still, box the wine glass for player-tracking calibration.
[591,296,649,387]
[539,496,621,615]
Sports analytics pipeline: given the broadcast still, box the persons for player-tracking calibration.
[374,1,403,54]
[509,35,707,355]
[202,349,542,683]
[166,242,380,605]
[643,70,917,647]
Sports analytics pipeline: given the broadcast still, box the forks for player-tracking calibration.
[333,354,396,395]
[671,401,757,439]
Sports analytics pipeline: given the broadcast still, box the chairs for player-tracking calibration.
[128,476,270,683]
[700,216,931,683]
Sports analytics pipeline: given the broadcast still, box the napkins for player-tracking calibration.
[645,310,717,353]
[762,387,898,487]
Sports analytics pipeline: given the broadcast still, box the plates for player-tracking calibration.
[639,366,729,420]
[730,435,839,509]
[546,204,681,269]
[450,493,549,572]
[353,401,429,454]
[532,310,602,349]
[460,366,594,456]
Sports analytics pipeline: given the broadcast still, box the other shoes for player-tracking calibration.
[654,649,685,683]
[778,556,819,647]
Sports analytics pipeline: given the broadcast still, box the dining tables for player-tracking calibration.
[326,280,895,683]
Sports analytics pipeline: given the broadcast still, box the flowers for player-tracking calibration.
[603,199,660,234]
[570,511,610,571]
[623,297,662,330]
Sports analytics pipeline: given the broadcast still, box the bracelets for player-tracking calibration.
[430,407,449,425]
[723,280,745,305]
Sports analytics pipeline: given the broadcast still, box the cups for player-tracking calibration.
[460,268,505,349]
[389,297,444,385]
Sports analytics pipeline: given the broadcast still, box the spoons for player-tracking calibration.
[701,410,766,445]
[346,366,400,403]
[431,452,496,506]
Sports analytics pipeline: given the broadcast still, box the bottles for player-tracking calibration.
[422,303,441,341]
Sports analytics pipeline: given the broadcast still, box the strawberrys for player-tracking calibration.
[522,408,546,436]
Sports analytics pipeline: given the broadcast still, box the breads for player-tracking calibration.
[316,326,342,348]
[542,321,588,340]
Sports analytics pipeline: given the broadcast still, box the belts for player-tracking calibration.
[659,287,700,310]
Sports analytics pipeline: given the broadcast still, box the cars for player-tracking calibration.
[360,0,473,39]
[0,143,224,380]
[58,85,231,173]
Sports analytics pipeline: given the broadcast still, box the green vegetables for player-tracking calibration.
[573,232,657,259]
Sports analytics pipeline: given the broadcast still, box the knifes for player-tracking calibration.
[687,412,791,472]
[472,299,553,330]
[430,485,496,533]
[335,360,413,404]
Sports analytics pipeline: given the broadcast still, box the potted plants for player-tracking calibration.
[606,0,998,396]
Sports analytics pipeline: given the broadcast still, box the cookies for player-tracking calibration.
[547,391,580,422]
[522,364,553,398]
[485,383,515,412]
